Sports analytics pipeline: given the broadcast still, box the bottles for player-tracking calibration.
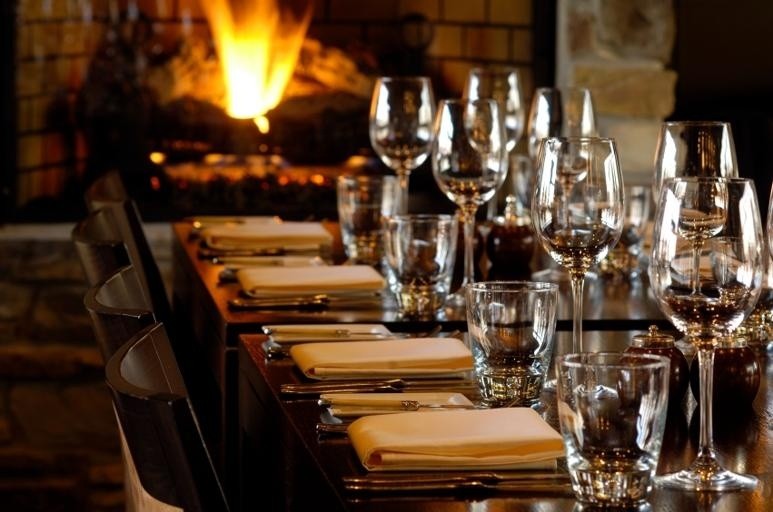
[625,328,688,442]
[685,402,757,471]
[691,330,761,420]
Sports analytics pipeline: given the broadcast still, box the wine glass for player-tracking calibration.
[650,119,739,295]
[429,99,513,315]
[527,84,600,218]
[532,136,623,350]
[461,66,522,228]
[651,175,763,490]
[372,72,432,264]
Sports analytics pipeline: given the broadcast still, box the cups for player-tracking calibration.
[467,280,551,407]
[554,349,670,510]
[596,182,650,278]
[387,217,458,315]
[337,175,398,261]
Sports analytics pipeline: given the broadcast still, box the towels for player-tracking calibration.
[239,262,386,301]
[289,336,476,385]
[347,408,569,475]
[203,218,334,253]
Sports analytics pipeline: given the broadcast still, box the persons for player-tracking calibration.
[75,10,155,177]
[660,1,773,242]
[364,10,480,215]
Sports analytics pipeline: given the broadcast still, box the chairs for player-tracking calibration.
[70,168,232,512]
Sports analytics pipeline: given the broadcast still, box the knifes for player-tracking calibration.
[344,469,570,490]
[229,292,386,312]
[274,381,469,396]
[266,328,392,338]
[316,398,502,411]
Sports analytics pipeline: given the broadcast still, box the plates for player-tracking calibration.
[262,323,394,347]
[319,389,475,417]
[223,255,327,272]
